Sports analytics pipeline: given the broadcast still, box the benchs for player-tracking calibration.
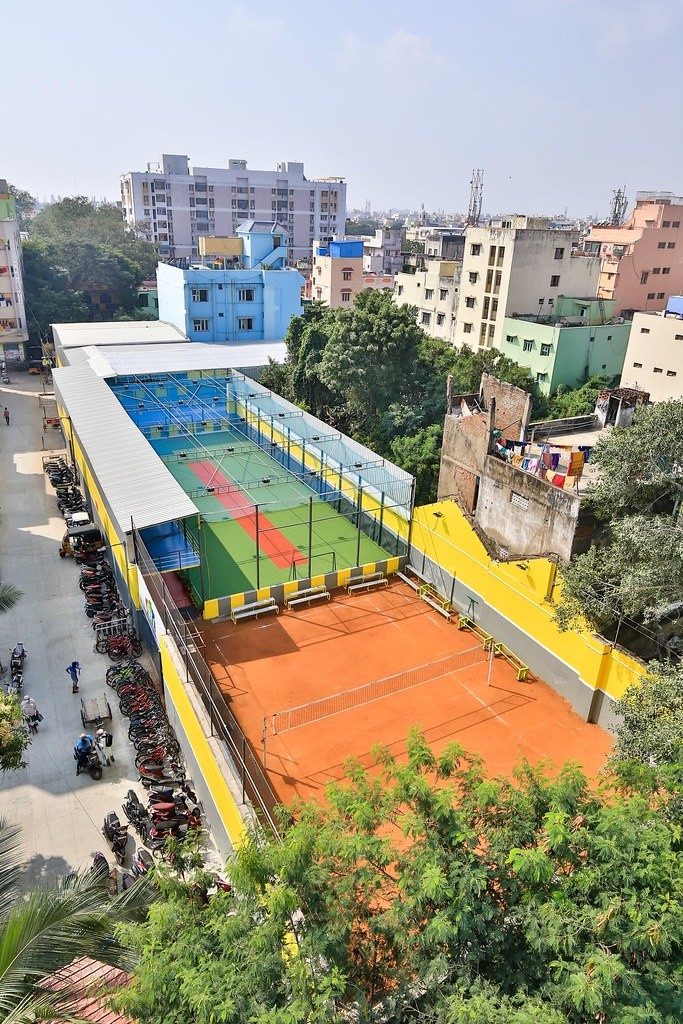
[229,564,451,626]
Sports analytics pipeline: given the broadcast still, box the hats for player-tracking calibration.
[96,729,104,734]
[24,695,30,699]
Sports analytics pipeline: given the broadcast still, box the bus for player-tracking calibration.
[27,346,55,374]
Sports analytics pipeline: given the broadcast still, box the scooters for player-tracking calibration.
[78,557,126,630]
[194,875,233,907]
[101,810,129,866]
[4,642,27,695]
[138,760,204,841]
[43,456,90,528]
[73,746,103,781]
[91,847,156,892]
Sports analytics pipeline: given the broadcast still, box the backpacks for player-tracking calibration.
[102,733,113,747]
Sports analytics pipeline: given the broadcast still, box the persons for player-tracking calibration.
[1,370,10,382]
[20,695,38,735]
[65,662,80,693]
[75,734,92,776]
[95,729,114,767]
[3,408,9,425]
[2,361,5,372]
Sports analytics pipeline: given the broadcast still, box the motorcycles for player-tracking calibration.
[122,789,150,845]
[58,524,103,558]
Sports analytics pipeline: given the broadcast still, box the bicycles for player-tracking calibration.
[95,627,180,768]
[152,831,194,861]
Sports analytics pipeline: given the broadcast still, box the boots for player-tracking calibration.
[103,759,110,766]
[76,686,79,690]
[73,687,78,693]
[110,755,114,762]
[76,769,79,776]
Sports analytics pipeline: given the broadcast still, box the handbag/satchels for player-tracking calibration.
[37,714,43,722]
